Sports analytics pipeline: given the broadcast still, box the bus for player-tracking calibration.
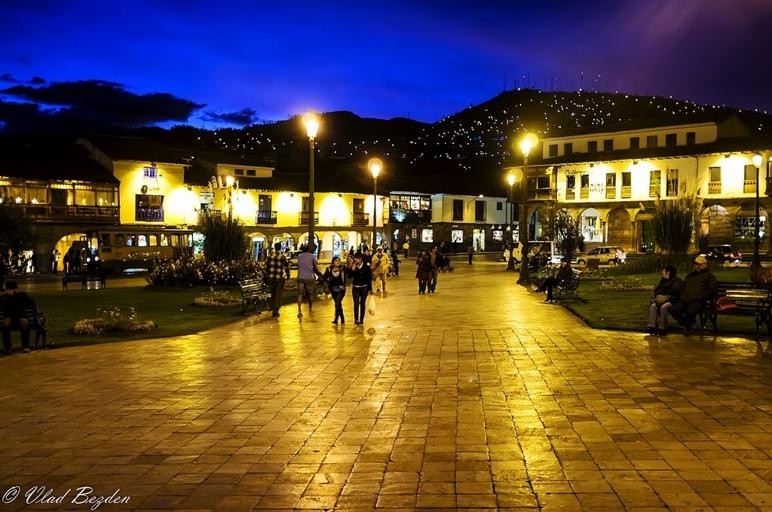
[97,228,197,275]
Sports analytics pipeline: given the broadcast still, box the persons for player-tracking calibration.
[670,256,719,335]
[10,249,61,273]
[263,237,474,325]
[647,265,683,329]
[0,280,31,355]
[533,257,573,304]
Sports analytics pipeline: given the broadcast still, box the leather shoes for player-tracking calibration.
[271,309,364,325]
[419,289,434,294]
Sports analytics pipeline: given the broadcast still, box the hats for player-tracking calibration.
[693,256,707,264]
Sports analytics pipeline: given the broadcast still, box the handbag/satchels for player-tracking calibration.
[656,295,670,305]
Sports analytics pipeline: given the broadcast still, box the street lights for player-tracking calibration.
[506,172,517,270]
[517,139,532,284]
[750,150,764,283]
[367,156,384,250]
[304,112,321,249]
[224,175,234,226]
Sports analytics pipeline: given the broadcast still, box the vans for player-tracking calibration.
[503,239,556,262]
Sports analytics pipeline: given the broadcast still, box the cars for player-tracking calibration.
[576,246,627,265]
[694,244,740,262]
[285,249,303,267]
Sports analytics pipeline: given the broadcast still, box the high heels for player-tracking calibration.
[544,298,553,302]
[535,289,542,293]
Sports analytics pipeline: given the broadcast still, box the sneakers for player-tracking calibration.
[647,326,658,335]
[681,318,696,325]
[657,329,668,336]
[684,321,693,333]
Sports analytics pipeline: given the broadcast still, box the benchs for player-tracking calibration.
[697,283,771,335]
[1,290,51,351]
[548,268,588,305]
[238,279,272,314]
